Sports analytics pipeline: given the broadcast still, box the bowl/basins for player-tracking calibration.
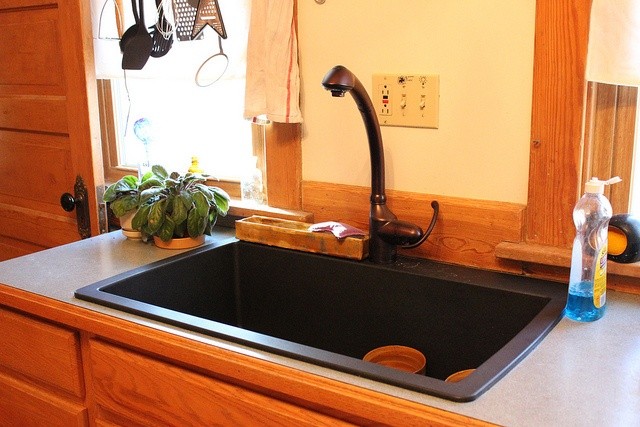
[363,346,426,378]
[445,369,477,383]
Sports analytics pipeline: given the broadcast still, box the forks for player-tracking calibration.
[152,0,174,53]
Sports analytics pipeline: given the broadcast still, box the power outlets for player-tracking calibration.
[371,72,440,131]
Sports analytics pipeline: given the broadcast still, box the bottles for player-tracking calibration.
[241,156,263,207]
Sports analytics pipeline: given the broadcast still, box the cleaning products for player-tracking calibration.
[563,176,623,325]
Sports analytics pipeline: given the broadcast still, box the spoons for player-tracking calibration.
[120,0,140,52]
[134,118,152,167]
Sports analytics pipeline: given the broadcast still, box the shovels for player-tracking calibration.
[122,0,153,70]
[150,0,173,57]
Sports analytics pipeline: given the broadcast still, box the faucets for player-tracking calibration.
[323,64,422,263]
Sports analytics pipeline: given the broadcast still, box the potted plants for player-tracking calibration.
[102,173,152,240]
[132,164,230,249]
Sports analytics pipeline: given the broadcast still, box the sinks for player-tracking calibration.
[73,236,568,403]
